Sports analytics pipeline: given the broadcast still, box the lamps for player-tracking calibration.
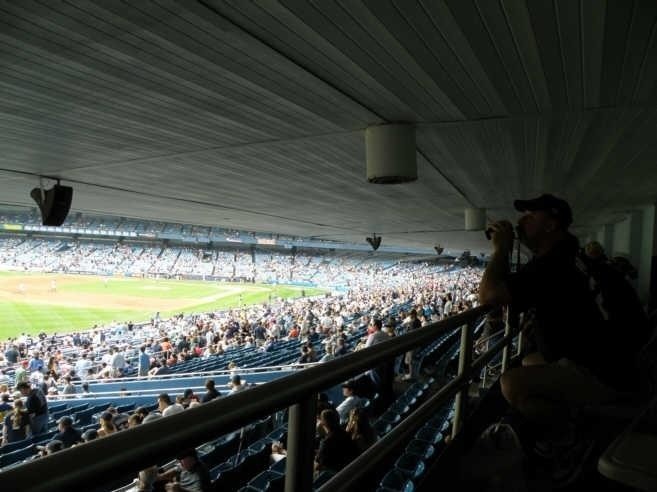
[30,171,76,229]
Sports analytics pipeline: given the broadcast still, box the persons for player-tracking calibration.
[0,194,657,487]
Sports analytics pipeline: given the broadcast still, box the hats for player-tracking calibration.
[55,415,73,424]
[513,193,573,225]
[340,380,354,387]
[97,411,112,419]
[80,428,96,439]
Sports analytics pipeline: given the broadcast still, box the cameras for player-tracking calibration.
[485,221,515,240]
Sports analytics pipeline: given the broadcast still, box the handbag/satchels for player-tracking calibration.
[456,422,528,491]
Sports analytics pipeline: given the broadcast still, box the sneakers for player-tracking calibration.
[533,433,597,489]
